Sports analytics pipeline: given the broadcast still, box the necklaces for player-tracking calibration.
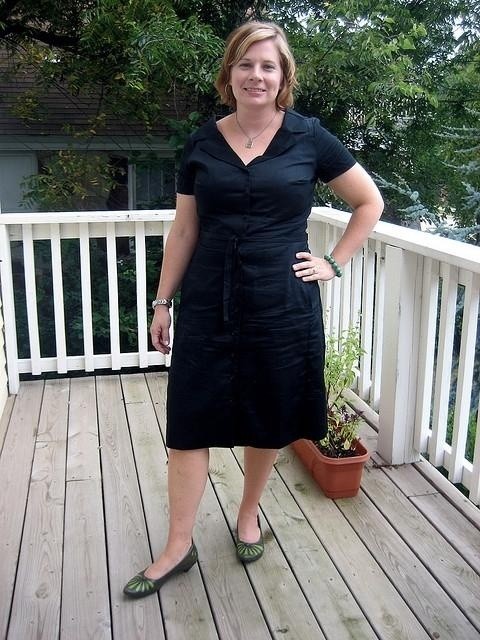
[233,109,279,149]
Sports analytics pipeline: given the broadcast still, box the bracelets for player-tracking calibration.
[150,299,174,309]
[323,255,343,278]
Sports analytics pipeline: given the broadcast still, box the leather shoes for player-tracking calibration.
[237,514,264,563]
[122,540,197,598]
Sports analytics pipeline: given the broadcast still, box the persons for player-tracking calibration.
[120,21,386,602]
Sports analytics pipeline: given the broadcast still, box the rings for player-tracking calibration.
[311,269,317,275]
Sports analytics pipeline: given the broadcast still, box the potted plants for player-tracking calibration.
[283,302,369,499]
